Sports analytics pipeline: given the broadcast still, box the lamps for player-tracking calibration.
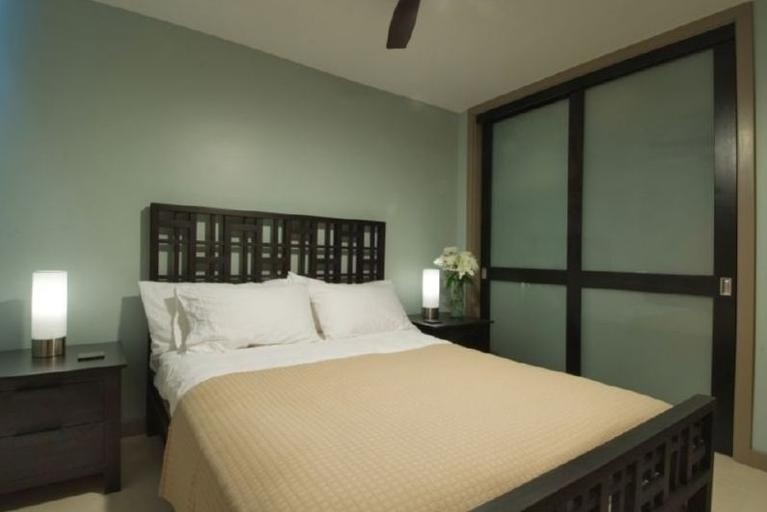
[28,268,71,360]
[421,268,439,323]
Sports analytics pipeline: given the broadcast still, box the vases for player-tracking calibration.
[448,278,465,319]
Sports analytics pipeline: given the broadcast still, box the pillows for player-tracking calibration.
[172,277,322,350]
[310,280,424,343]
[136,274,188,355]
[286,271,326,337]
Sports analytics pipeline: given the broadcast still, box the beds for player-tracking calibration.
[144,198,720,512]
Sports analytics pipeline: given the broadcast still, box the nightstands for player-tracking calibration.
[1,340,128,505]
[407,308,498,356]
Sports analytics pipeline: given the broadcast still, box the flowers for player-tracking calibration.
[433,246,479,314]
